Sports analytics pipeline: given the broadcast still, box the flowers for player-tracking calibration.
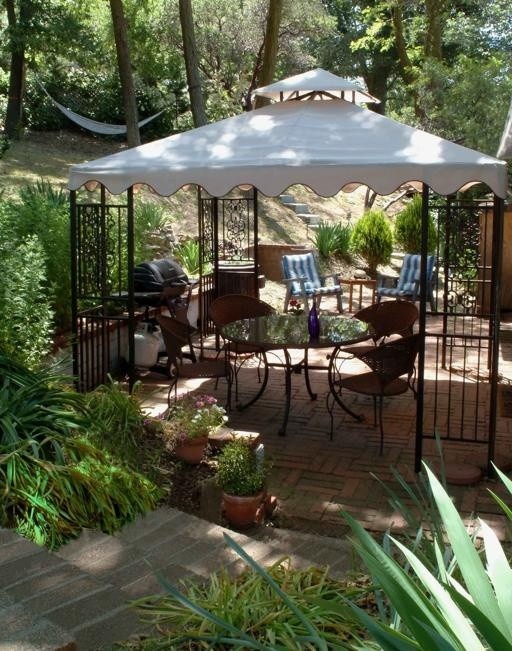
[163,393,226,445]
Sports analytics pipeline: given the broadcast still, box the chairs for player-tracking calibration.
[327,331,425,441]
[281,251,343,312]
[157,315,234,418]
[375,254,436,311]
[332,301,423,396]
[208,293,276,387]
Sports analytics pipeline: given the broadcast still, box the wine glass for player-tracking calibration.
[318,311,328,340]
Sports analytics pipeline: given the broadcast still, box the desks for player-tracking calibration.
[336,277,377,313]
[221,314,375,437]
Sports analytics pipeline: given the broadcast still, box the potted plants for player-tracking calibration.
[217,430,272,532]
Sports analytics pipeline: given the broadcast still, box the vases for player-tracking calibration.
[171,435,208,468]
[306,304,317,339]
[287,301,303,321]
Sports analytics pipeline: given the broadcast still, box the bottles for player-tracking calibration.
[308,295,320,339]
